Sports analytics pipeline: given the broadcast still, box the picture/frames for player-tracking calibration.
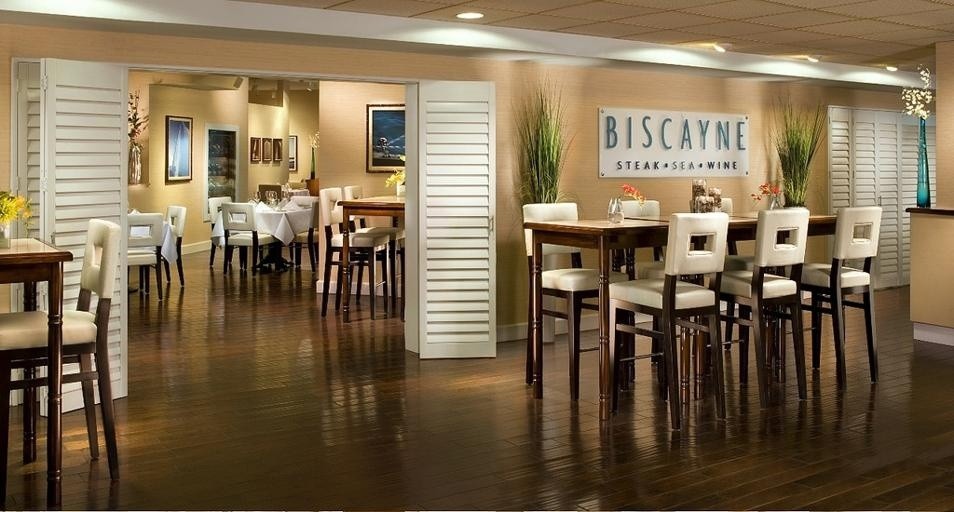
[165,115,193,183]
[203,122,240,221]
[273,139,283,161]
[288,135,298,172]
[366,103,405,173]
[250,137,261,162]
[262,137,273,162]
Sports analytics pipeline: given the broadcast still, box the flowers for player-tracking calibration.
[900,68,935,119]
[310,130,320,149]
[385,155,405,188]
[128,89,149,148]
[750,184,782,201]
[621,184,646,206]
[0,189,33,232]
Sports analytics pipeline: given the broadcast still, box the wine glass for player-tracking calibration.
[252,183,291,209]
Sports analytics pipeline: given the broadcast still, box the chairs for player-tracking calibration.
[208,179,405,323]
[127,206,186,299]
[0,217,122,511]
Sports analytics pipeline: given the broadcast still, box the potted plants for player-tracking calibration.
[510,74,573,344]
[766,86,829,276]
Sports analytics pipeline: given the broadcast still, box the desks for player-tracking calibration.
[0,237,74,491]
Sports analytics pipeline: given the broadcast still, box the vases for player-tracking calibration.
[310,147,315,180]
[917,118,930,207]
[129,149,142,184]
[0,234,10,248]
